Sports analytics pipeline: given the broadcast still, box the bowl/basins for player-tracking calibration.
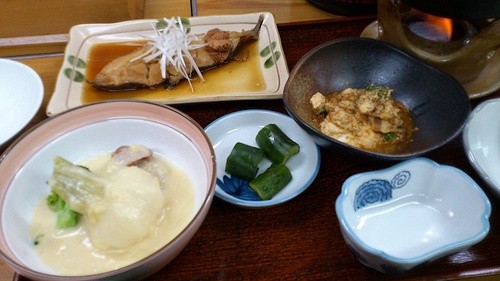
[283,36,470,160]
[335,156,492,271]
[0,101,217,281]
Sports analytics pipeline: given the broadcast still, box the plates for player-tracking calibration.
[360,19,500,99]
[0,57,45,146]
[463,98,500,194]
[46,12,290,119]
[204,110,320,207]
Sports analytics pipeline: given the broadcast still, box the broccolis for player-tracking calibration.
[321,83,399,143]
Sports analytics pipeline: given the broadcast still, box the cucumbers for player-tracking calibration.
[224,124,300,200]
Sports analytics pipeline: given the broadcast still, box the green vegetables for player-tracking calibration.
[45,159,90,228]
